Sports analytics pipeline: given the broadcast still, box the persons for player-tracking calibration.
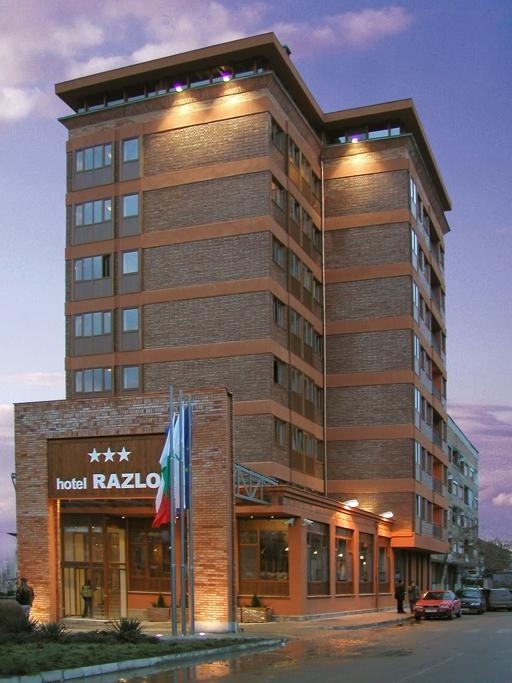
[81,580,98,618]
[393,578,407,613]
[406,579,420,613]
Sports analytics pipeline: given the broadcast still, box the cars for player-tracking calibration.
[457,586,488,614]
[479,587,512,611]
[415,588,462,619]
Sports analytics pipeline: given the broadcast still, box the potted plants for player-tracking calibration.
[239,592,273,623]
[148,590,190,624]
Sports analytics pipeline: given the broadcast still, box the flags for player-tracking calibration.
[150,404,189,529]
